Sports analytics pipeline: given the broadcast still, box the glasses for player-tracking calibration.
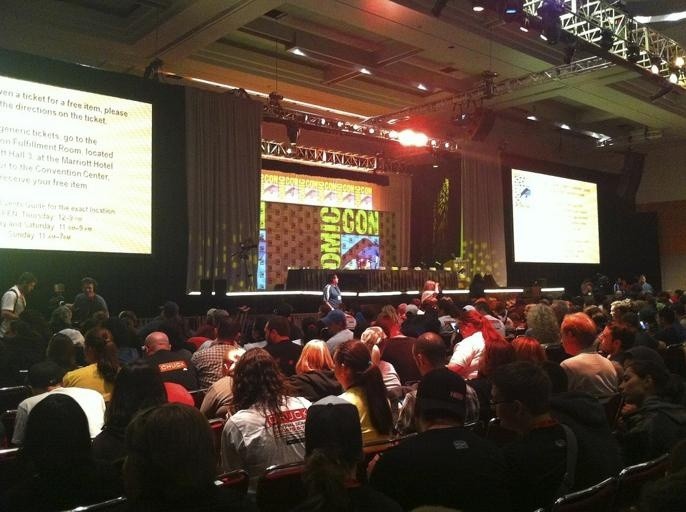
[487,397,516,411]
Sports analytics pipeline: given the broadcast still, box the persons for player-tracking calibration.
[1,273,685,510]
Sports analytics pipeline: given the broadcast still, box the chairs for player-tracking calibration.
[258,460,309,511]
[0,385,34,409]
[362,437,397,466]
[554,475,618,511]
[213,468,249,511]
[618,454,671,497]
[72,496,127,512]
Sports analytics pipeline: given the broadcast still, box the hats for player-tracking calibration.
[305,395,363,455]
[417,366,466,414]
[320,310,347,327]
[159,302,180,312]
[24,360,66,387]
